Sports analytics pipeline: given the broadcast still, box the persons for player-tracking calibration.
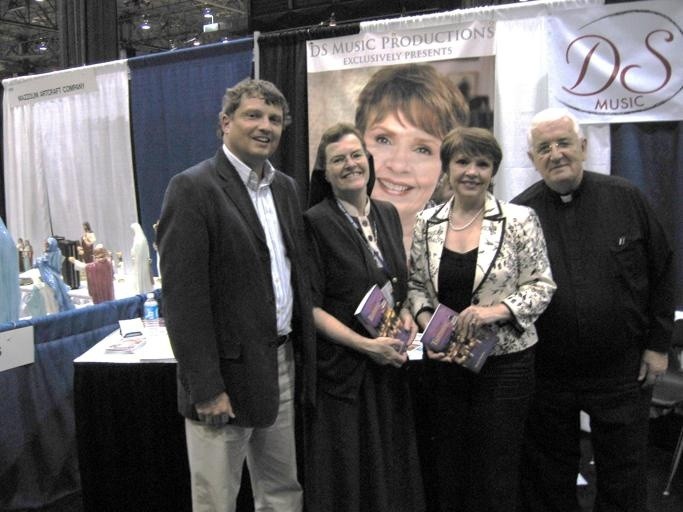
[407,128,558,511]
[355,62,472,267]
[156,75,316,512]
[509,107,677,512]
[68,244,116,306]
[16,237,22,253]
[77,222,96,264]
[301,121,427,512]
[130,223,154,295]
[24,239,33,267]
[31,237,75,312]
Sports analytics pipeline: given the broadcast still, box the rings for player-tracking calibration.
[469,321,476,328]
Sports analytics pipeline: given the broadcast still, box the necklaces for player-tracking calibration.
[447,205,487,231]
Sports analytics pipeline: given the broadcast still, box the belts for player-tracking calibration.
[274,331,295,350]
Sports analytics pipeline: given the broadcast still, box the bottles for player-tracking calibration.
[144,292,160,337]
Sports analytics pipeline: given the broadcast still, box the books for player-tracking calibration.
[103,337,148,355]
[354,282,412,357]
[419,302,499,375]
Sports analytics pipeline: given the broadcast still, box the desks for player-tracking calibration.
[71,319,190,510]
